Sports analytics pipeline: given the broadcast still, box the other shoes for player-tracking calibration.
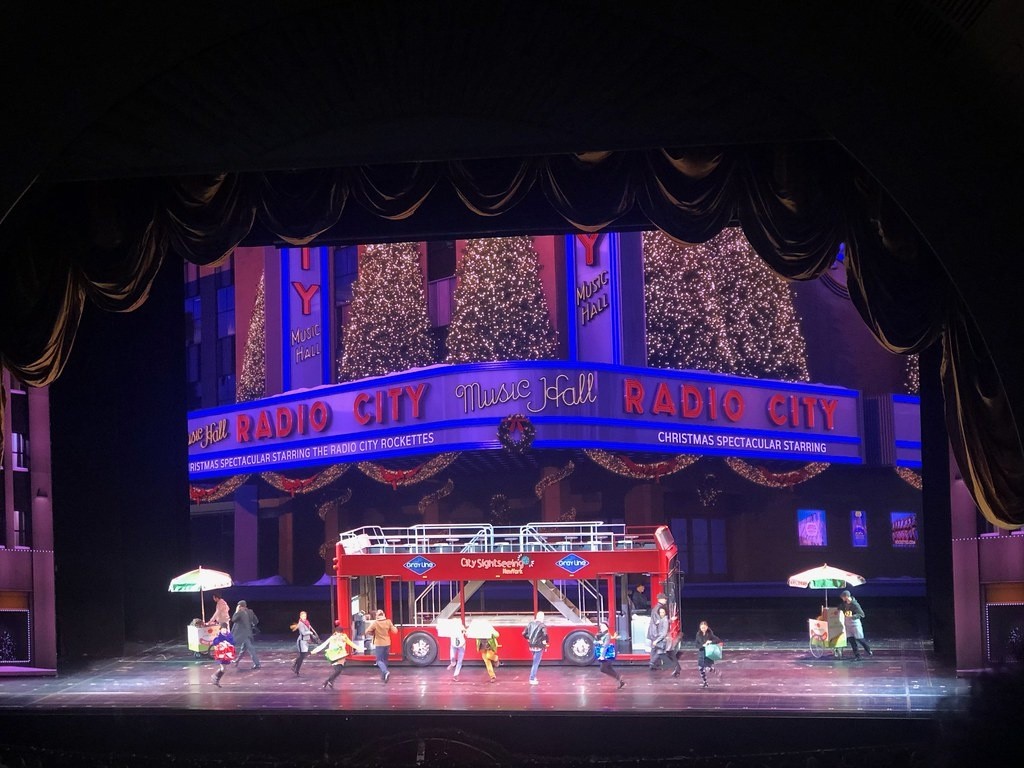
[672,667,682,677]
[291,666,302,677]
[854,657,861,661]
[232,660,238,666]
[530,680,538,685]
[321,681,335,689]
[491,677,496,683]
[382,672,390,683]
[701,683,708,688]
[616,682,627,690]
[494,660,499,667]
[718,673,723,681]
[249,664,260,670]
[447,664,453,671]
[649,661,662,670]
[453,676,459,682]
[868,651,872,655]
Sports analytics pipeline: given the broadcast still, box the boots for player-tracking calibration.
[212,669,223,688]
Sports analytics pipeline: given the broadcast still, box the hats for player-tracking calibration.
[657,593,668,598]
[841,591,850,597]
[238,600,246,605]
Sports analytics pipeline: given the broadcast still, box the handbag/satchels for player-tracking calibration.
[537,631,549,649]
[705,643,722,661]
[310,633,319,644]
[595,644,616,659]
[655,641,667,649]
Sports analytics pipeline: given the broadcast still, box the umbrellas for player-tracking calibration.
[786,562,867,608]
[168,565,233,623]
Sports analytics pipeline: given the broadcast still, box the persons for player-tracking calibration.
[311,627,367,689]
[205,594,230,636]
[593,621,627,690]
[435,624,466,677]
[522,612,549,684]
[477,631,500,683]
[695,620,723,687]
[839,590,873,661]
[289,611,313,677]
[627,583,648,615]
[210,622,234,689]
[647,592,682,678]
[365,609,398,684]
[232,599,261,670]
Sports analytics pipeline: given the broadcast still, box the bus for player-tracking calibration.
[331,520,684,667]
